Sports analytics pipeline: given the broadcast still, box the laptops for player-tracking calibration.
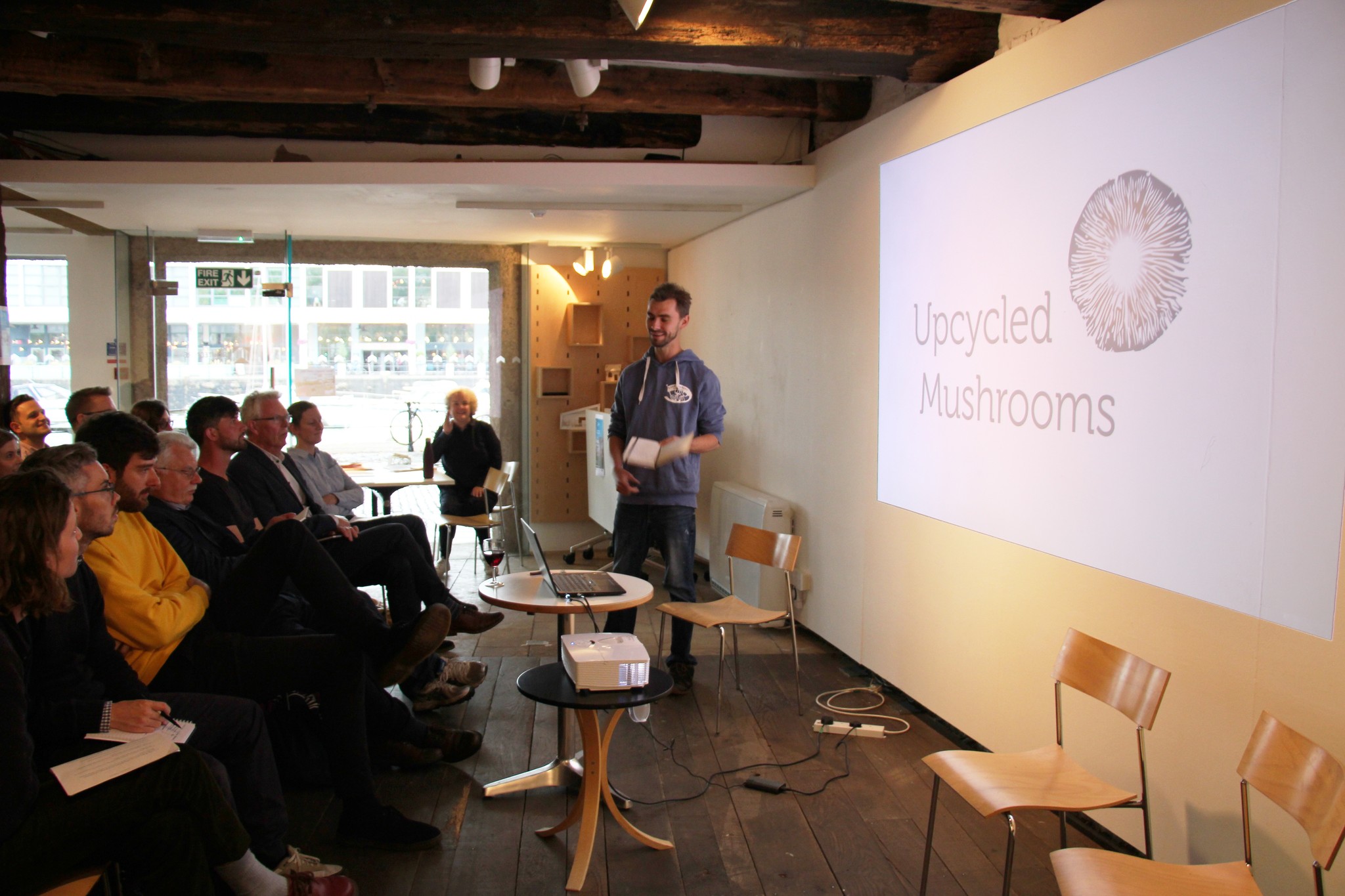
[521,519,627,596]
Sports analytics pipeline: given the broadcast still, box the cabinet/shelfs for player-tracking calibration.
[567,302,604,347]
[629,336,650,362]
[600,381,617,411]
[537,366,572,399]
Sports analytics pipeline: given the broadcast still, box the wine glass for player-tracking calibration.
[483,538,505,587]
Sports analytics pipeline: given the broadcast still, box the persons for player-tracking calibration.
[0,388,506,896]
[602,282,726,693]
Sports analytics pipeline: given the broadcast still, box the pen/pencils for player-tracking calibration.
[154,710,182,730]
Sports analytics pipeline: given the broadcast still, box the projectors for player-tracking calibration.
[561,632,650,690]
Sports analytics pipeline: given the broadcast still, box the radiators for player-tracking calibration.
[709,479,794,629]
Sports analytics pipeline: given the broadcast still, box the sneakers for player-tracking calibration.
[413,679,474,713]
[437,659,488,689]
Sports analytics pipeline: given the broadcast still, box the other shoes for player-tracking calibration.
[435,558,449,578]
[668,668,693,694]
[446,606,504,637]
[418,721,483,763]
[376,602,451,687]
[437,639,455,653]
[273,845,344,876]
[480,553,498,576]
[381,737,443,767]
[340,805,442,851]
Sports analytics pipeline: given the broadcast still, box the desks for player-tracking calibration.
[331,449,456,518]
[516,660,674,892]
[477,569,654,812]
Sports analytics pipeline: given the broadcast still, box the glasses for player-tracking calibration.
[158,418,171,429]
[72,482,116,497]
[252,413,293,426]
[160,465,202,479]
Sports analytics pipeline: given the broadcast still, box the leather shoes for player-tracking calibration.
[280,869,357,896]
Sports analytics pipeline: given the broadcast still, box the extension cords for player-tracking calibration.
[814,719,887,740]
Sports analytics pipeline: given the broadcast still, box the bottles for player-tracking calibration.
[423,438,434,479]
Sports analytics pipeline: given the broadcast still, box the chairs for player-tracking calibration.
[919,627,1172,896]
[654,524,801,736]
[1050,709,1345,896]
[431,461,524,589]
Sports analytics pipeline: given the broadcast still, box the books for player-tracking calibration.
[84,716,196,745]
[621,429,694,470]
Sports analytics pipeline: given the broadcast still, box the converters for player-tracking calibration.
[746,777,786,793]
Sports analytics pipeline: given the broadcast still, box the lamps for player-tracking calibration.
[468,58,516,90]
[564,58,608,98]
[600,247,624,281]
[617,0,654,31]
[572,248,596,276]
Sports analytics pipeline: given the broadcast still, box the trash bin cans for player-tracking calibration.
[294,367,337,397]
